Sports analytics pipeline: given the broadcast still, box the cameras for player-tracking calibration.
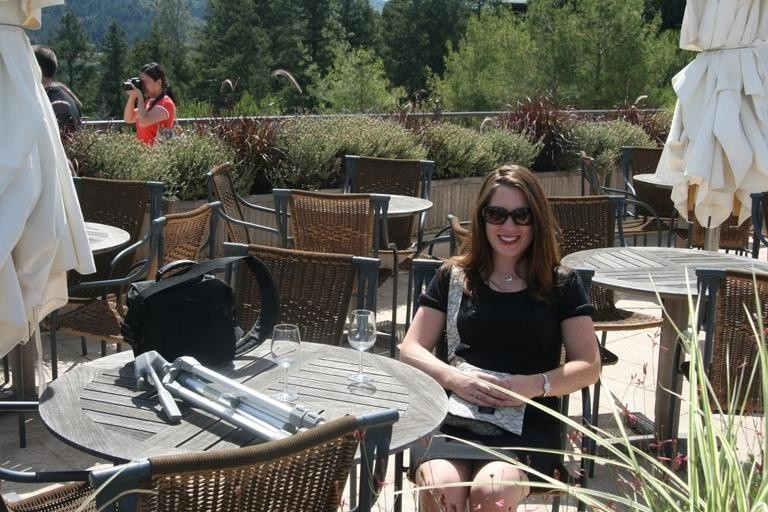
[123,77,144,91]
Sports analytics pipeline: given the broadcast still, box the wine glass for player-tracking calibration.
[271,323,302,401]
[347,309,377,385]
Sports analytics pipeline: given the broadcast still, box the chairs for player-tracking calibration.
[272,188,400,359]
[205,161,291,285]
[667,185,751,258]
[48,200,222,381]
[393,319,619,512]
[548,195,663,478]
[580,150,662,247]
[221,241,381,353]
[0,408,399,512]
[665,266,768,470]
[72,176,165,355]
[621,146,691,247]
[341,154,435,270]
[406,215,475,335]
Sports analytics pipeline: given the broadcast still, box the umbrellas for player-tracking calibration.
[0,0,96,400]
[654,0,768,253]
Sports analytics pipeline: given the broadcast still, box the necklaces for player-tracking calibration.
[488,261,527,285]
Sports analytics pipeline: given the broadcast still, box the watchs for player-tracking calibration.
[541,371,552,400]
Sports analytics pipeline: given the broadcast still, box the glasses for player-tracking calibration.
[482,206,532,225]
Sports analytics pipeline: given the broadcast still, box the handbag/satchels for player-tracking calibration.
[126,255,281,368]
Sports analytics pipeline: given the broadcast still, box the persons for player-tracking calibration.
[124,63,176,148]
[27,43,80,132]
[399,164,601,512]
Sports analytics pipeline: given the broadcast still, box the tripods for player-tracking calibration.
[129,350,325,445]
